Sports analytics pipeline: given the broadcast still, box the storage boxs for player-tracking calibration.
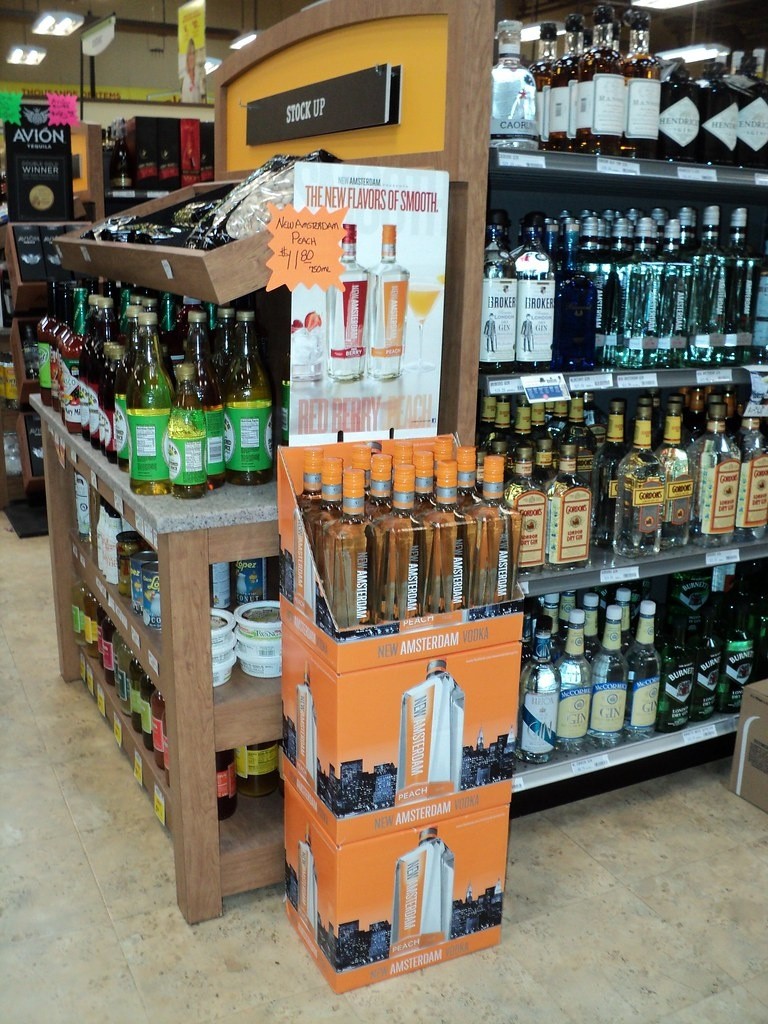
[3,218,94,310]
[276,433,524,674]
[14,412,47,490]
[10,317,49,402]
[126,116,214,155]
[729,680,768,815]
[282,780,511,993]
[282,622,522,846]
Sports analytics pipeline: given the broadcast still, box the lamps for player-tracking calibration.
[32,9,85,37]
[228,1,261,50]
[205,57,222,76]
[5,0,47,66]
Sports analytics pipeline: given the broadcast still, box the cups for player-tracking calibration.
[291,317,324,380]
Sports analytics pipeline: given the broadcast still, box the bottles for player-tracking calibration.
[109,130,214,191]
[390,826,455,961]
[298,821,318,940]
[479,204,768,375]
[95,495,136,584]
[393,657,465,808]
[70,575,284,822]
[488,6,768,169]
[298,435,524,628]
[476,386,767,576]
[297,660,317,794]
[515,556,768,766]
[38,272,290,500]
[365,224,410,380]
[324,223,369,382]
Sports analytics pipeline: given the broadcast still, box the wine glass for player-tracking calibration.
[404,283,444,374]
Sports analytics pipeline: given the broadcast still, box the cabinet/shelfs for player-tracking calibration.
[0,0,768,926]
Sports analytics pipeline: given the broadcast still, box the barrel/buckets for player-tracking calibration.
[117,531,268,630]
